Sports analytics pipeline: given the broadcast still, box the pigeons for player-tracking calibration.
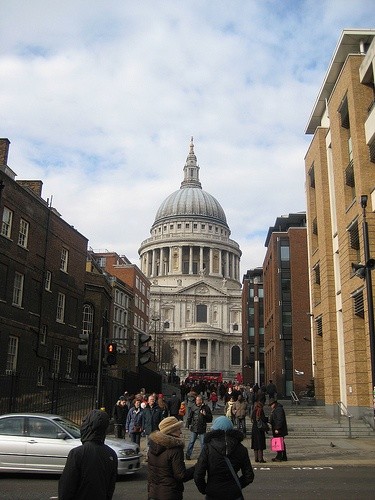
[328,441,337,448]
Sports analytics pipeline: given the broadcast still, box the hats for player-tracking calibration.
[158,417,184,434]
[268,399,277,406]
[211,416,233,431]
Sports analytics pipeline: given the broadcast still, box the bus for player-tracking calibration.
[188,371,223,383]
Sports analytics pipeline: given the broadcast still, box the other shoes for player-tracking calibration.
[272,456,288,462]
[256,460,266,463]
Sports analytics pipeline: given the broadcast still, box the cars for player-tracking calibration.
[0,412,145,477]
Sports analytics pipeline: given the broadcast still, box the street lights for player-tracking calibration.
[151,317,162,362]
[351,193,375,391]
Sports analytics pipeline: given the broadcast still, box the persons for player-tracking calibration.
[32,421,45,436]
[58,410,117,500]
[194,415,255,500]
[145,417,194,500]
[112,373,287,462]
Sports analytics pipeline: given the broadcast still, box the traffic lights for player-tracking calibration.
[134,333,152,369]
[76,332,97,369]
[103,342,118,366]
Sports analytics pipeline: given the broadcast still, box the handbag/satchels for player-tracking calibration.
[256,417,270,432]
[270,437,285,452]
